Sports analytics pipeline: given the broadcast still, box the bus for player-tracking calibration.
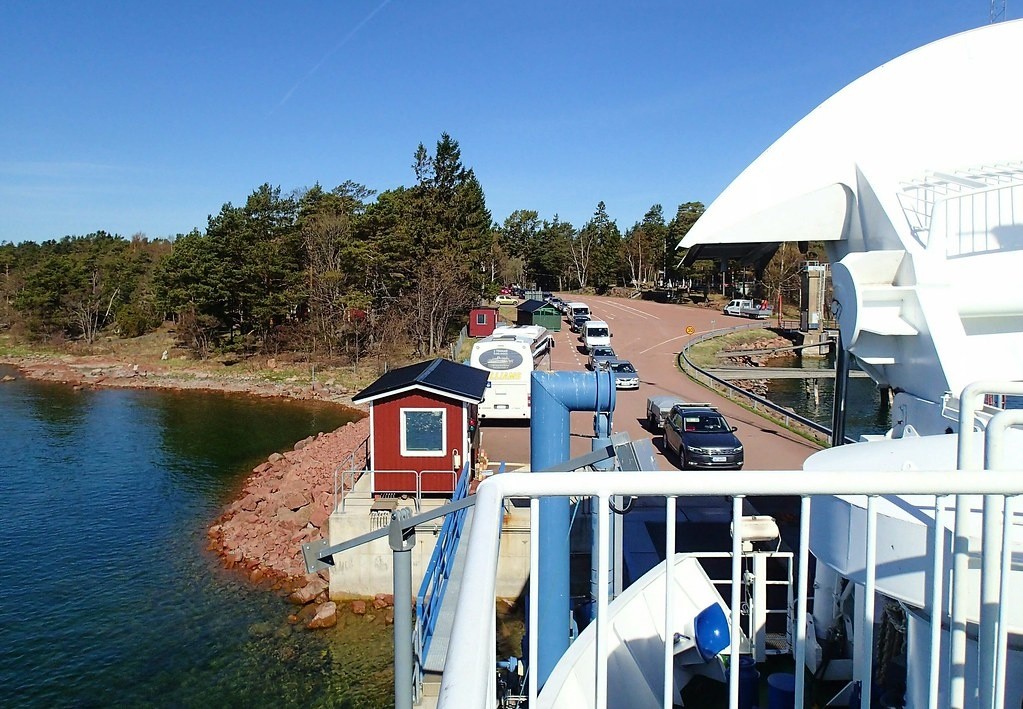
[471,323,556,420]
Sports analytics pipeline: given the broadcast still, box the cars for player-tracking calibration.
[500,286,572,315]
[589,346,619,371]
[606,360,640,389]
[571,316,592,332]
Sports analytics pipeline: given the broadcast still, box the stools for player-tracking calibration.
[767,672,807,709]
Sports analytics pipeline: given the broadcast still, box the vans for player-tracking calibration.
[567,303,593,320]
[583,321,615,355]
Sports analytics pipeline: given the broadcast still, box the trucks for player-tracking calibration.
[722,298,772,320]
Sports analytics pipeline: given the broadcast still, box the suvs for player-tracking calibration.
[664,402,745,471]
